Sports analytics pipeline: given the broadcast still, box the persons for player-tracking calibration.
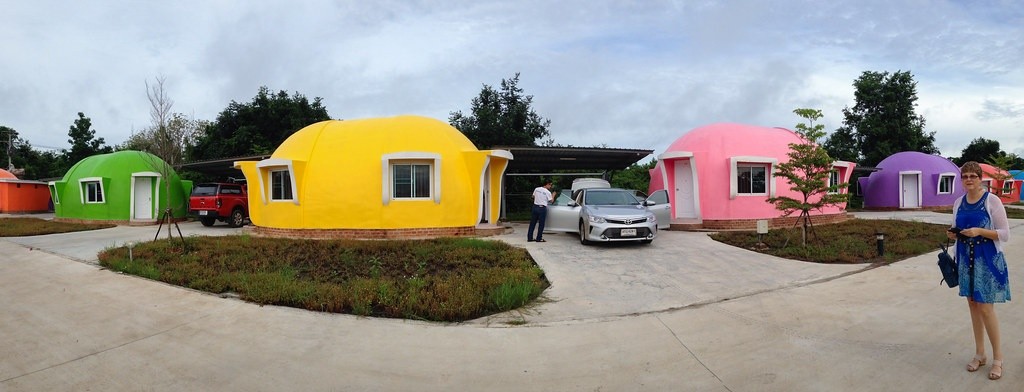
[527,178,557,242]
[946,161,1011,380]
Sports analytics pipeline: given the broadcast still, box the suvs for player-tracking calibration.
[188,181,250,227]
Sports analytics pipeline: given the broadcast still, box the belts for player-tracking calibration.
[958,237,988,294]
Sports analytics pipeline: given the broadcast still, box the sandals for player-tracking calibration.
[967,353,987,372]
[988,358,1003,380]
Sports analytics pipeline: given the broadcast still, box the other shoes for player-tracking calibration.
[537,239,545,242]
[528,238,536,241]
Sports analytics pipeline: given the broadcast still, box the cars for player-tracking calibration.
[542,178,672,245]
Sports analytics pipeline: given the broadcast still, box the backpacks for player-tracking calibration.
[937,237,962,288]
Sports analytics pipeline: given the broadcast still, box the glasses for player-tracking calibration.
[960,175,980,180]
